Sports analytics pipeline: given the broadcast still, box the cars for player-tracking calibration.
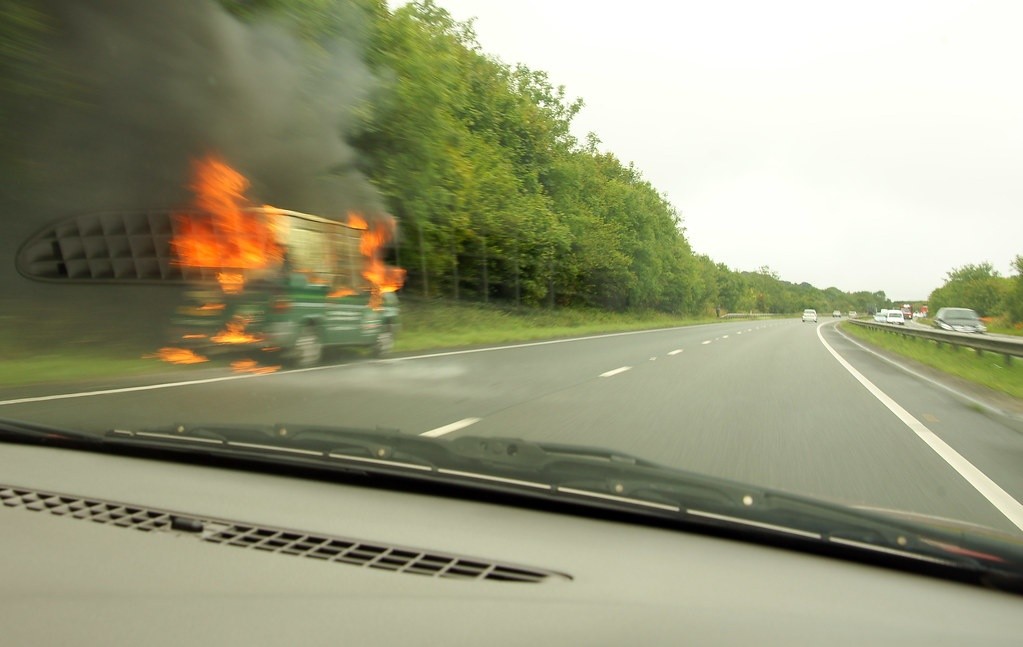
[849,311,857,318]
[872,308,926,325]
[833,310,841,318]
[802,309,817,322]
[931,307,987,335]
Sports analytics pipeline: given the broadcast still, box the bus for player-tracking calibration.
[178,207,401,367]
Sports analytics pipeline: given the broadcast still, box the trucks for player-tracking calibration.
[900,304,913,319]
